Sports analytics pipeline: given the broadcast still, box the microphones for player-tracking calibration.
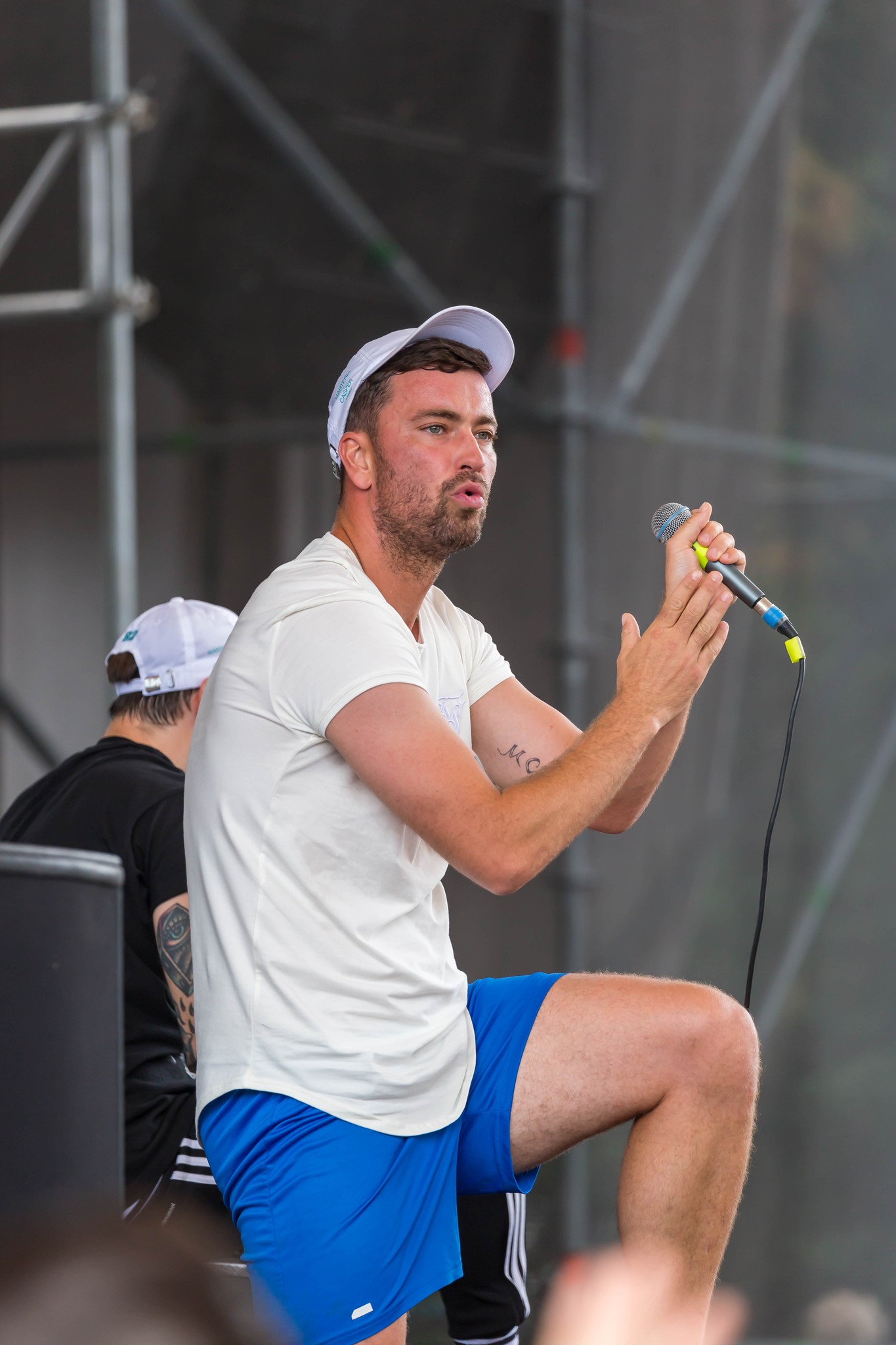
[652,502,798,639]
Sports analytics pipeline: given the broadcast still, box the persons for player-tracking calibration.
[0,597,532,1345]
[184,306,762,1345]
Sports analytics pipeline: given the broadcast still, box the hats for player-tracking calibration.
[104,596,239,695]
[327,305,516,479]
